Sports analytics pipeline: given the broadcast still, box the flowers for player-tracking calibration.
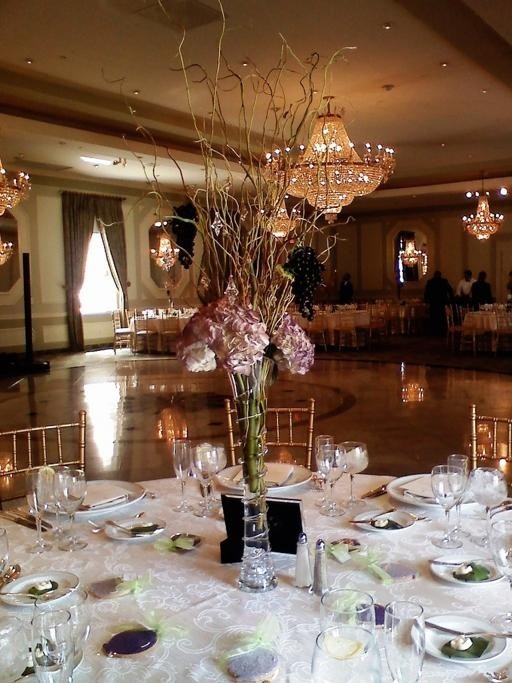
[173,296,323,533]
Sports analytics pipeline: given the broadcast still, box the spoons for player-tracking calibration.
[346,508,396,524]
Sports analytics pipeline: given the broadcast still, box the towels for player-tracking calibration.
[397,475,437,502]
[232,465,295,487]
[68,483,130,512]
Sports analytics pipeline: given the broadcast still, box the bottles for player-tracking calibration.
[314,539,329,596]
[293,533,313,588]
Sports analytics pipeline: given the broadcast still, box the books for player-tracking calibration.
[221,492,307,554]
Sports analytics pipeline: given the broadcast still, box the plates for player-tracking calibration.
[386,469,430,507]
[428,555,506,588]
[29,478,147,515]
[103,516,166,542]
[427,615,509,666]
[352,507,419,534]
[216,462,312,491]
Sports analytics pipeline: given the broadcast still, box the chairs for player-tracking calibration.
[101,290,511,361]
[0,406,88,509]
[221,393,319,469]
[469,400,512,474]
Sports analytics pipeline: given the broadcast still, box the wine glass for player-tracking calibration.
[361,295,425,304]
[489,519,512,595]
[310,588,384,682]
[430,453,469,550]
[312,433,369,518]
[480,301,510,312]
[0,465,91,682]
[170,435,227,518]
[141,301,357,315]
[470,467,507,547]
[383,599,426,683]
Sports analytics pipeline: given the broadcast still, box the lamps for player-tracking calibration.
[460,166,506,242]
[256,155,301,235]
[261,91,398,228]
[149,220,180,273]
[0,155,32,267]
[397,232,422,267]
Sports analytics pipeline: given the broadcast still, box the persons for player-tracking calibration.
[506,271,511,310]
[337,271,354,303]
[423,269,454,337]
[454,269,477,304]
[471,270,496,310]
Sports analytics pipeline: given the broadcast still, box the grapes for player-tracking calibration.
[172,202,199,269]
[286,244,329,323]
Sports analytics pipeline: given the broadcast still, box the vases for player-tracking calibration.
[224,355,283,593]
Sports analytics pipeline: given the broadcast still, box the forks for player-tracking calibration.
[427,557,496,567]
[424,618,512,642]
[363,483,386,499]
